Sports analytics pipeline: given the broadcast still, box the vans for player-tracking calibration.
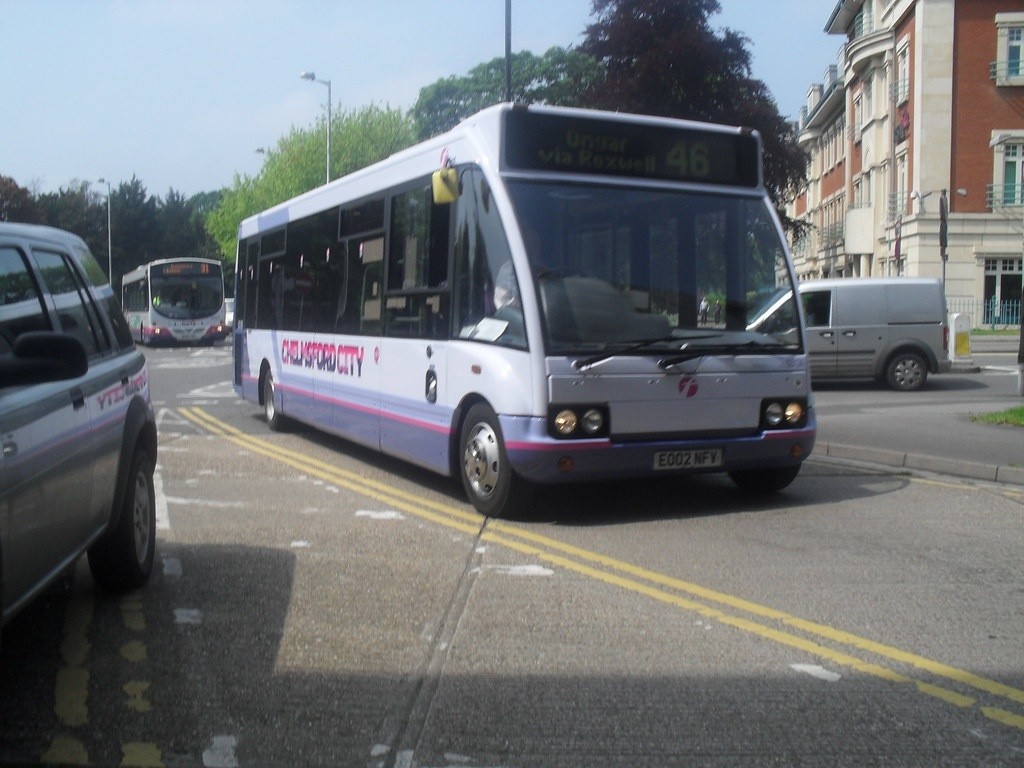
[1,222,159,635]
[745,276,952,392]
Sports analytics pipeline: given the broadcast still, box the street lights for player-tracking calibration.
[910,187,968,289]
[300,71,331,185]
[96,176,112,289]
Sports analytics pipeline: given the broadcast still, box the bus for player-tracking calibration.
[232,101,818,518]
[121,257,228,346]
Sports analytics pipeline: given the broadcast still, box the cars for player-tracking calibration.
[224,297,235,332]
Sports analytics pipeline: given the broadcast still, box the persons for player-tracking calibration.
[699,296,710,325]
[805,298,819,327]
[153,291,163,310]
[620,283,631,299]
[763,310,790,336]
[713,299,722,325]
[494,221,549,312]
